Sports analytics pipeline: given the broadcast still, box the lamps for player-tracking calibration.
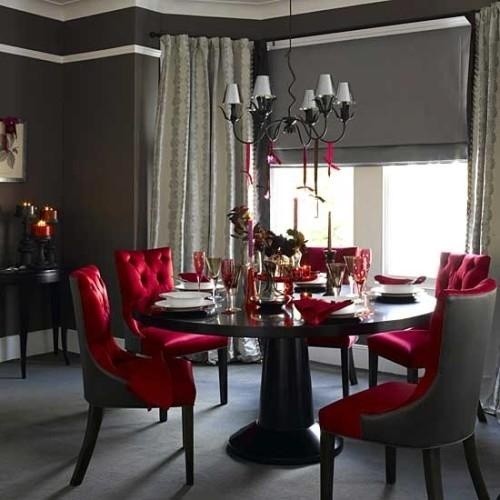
[218,0,356,148]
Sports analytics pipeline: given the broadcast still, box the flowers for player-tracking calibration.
[227,205,263,243]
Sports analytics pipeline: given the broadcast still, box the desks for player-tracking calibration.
[0,263,71,379]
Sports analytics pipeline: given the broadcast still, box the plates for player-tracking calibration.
[368,275,426,298]
[155,273,222,310]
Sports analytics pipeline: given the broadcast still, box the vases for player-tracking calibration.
[242,243,262,295]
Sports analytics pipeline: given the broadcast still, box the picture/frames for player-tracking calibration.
[0,117,27,183]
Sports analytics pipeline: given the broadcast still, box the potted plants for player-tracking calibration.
[264,229,307,278]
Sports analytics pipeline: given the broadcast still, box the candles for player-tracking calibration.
[294,199,298,230]
[328,210,331,250]
[16,201,58,237]
[248,219,254,260]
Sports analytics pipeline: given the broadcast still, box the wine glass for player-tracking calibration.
[193,250,222,310]
[220,257,241,316]
[325,248,370,303]
